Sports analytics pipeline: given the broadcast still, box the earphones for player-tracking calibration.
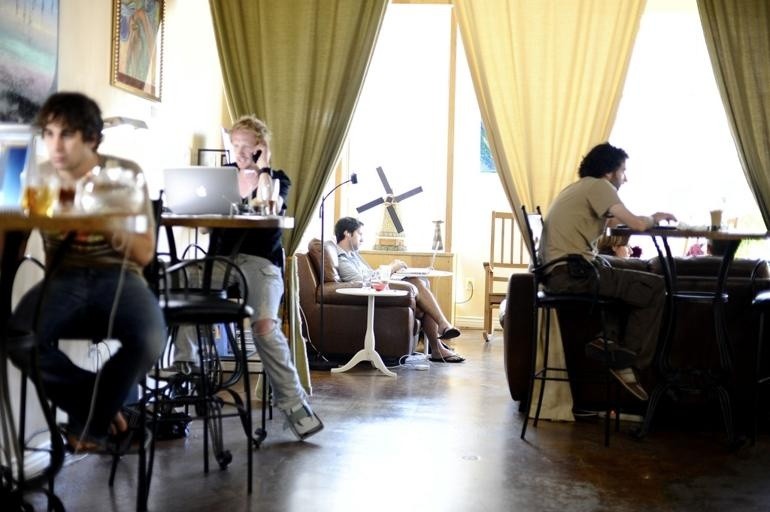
[348,233,349,237]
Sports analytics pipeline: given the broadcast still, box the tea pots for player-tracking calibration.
[73,156,149,215]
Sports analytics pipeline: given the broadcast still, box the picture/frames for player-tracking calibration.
[196,147,233,171]
[105,0,170,105]
[1,137,39,213]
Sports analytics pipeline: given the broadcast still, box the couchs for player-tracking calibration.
[497,255,770,436]
[294,236,424,372]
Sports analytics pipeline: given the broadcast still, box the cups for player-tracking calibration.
[379,265,392,289]
[259,176,280,214]
[710,208,723,230]
[21,157,60,218]
[361,268,373,289]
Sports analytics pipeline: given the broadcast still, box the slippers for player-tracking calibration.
[428,355,464,363]
[436,325,461,339]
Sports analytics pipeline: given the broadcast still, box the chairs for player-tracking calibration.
[743,254,770,447]
[0,188,298,512]
[518,204,631,450]
[482,209,536,343]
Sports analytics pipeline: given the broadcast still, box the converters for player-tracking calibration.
[411,362,429,370]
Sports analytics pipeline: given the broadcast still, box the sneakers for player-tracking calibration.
[607,363,650,403]
[281,399,325,442]
[584,336,615,359]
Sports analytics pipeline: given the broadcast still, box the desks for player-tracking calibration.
[609,224,770,453]
[329,286,410,379]
[375,263,456,361]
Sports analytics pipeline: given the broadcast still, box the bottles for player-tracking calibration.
[432,220,445,251]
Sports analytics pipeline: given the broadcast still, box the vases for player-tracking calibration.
[430,218,445,252]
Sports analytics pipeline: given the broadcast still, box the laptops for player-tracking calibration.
[162,167,240,215]
[0,133,34,213]
[396,240,439,274]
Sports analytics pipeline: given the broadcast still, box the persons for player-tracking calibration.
[596,230,635,260]
[330,214,467,364]
[5,91,173,455]
[158,113,324,442]
[535,136,678,418]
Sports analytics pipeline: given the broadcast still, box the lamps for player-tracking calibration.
[19,116,149,214]
[308,173,360,369]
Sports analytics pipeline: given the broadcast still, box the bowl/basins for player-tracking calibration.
[373,283,388,291]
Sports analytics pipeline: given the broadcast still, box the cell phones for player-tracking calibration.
[252,150,261,163]
